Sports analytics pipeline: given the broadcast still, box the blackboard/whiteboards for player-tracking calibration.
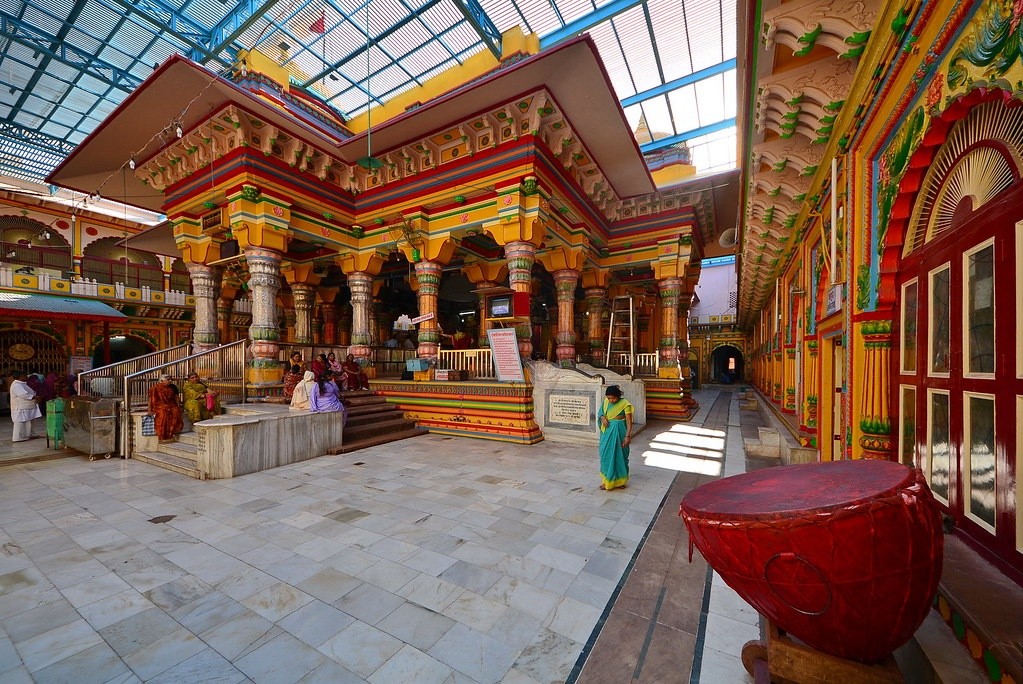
[486,328,525,383]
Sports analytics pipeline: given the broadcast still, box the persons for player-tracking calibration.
[282,364,304,405]
[311,354,331,382]
[341,353,369,392]
[9,370,42,442]
[597,384,635,491]
[289,370,317,412]
[281,351,308,382]
[182,372,223,426]
[147,374,184,441]
[326,353,349,392]
[0,367,102,416]
[309,370,347,427]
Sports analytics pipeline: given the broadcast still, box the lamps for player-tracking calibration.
[176,126,182,138]
[46,232,51,240]
[129,159,135,170]
[71,214,76,222]
[12,251,16,257]
[27,242,32,248]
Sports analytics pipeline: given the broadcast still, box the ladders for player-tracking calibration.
[605,294,637,380]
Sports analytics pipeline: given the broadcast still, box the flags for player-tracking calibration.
[309,16,324,33]
